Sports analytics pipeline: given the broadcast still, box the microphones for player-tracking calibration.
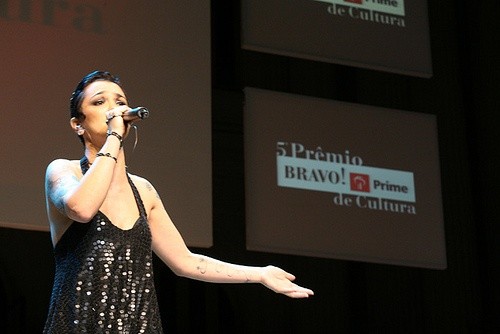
[105,106,150,127]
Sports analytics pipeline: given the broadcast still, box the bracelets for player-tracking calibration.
[106,131,123,149]
[97,153,117,163]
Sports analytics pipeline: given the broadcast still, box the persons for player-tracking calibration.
[43,72,314,334]
